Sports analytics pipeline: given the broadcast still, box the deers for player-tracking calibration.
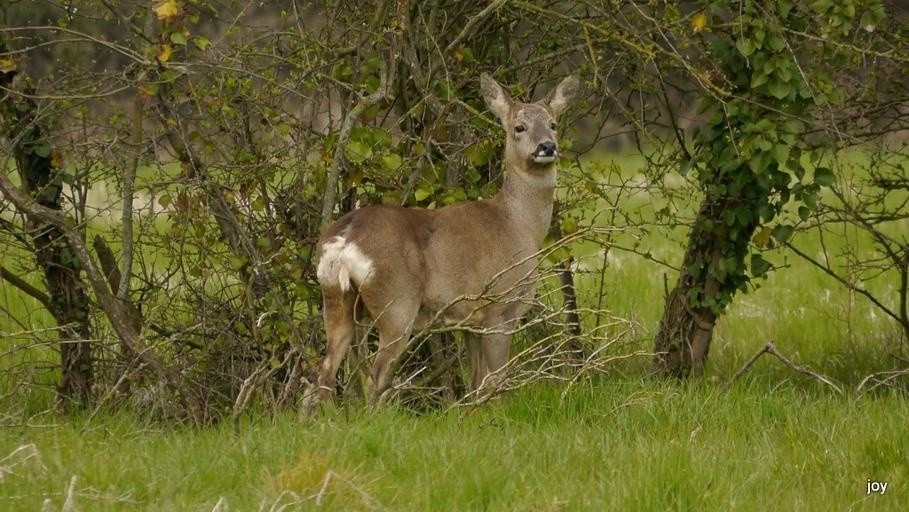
[299,68,582,426]
